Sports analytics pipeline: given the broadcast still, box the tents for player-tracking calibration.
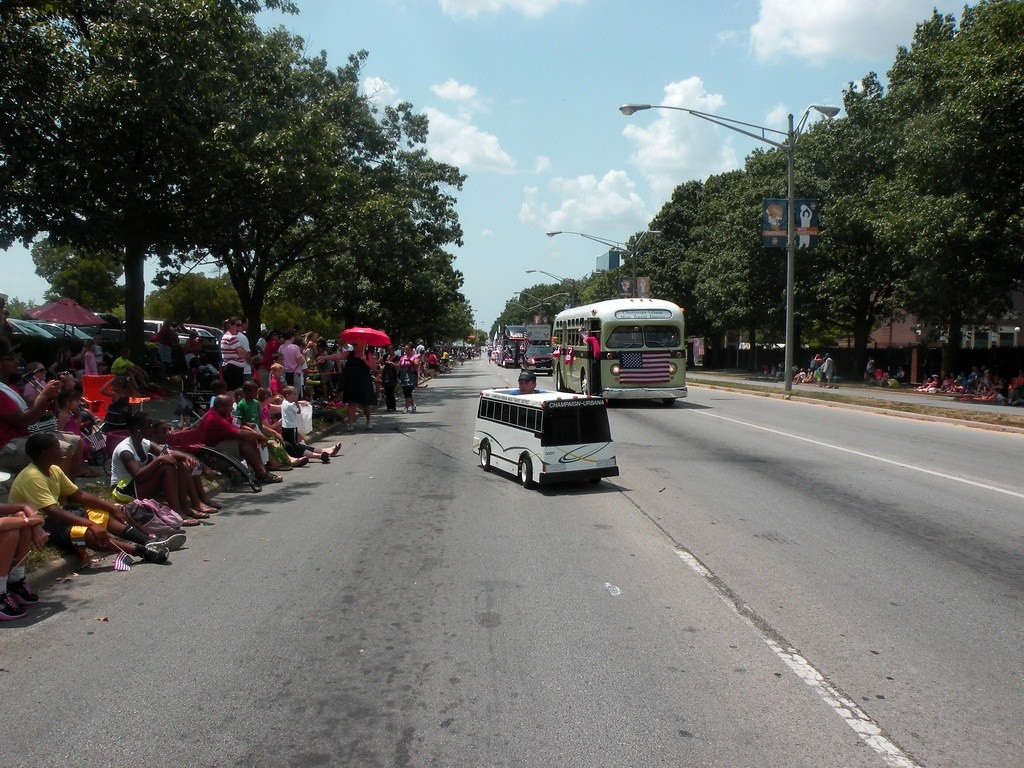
[451,338,473,348]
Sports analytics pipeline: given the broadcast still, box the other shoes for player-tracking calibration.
[347,425,353,431]
[792,380,798,385]
[412,405,416,412]
[319,452,330,463]
[402,407,408,413]
[330,443,341,457]
[179,501,221,525]
[367,422,373,429]
[293,456,308,467]
[385,409,393,414]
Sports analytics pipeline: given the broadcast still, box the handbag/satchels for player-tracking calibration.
[810,360,821,370]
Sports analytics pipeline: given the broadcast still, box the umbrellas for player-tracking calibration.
[339,326,392,354]
[26,298,110,344]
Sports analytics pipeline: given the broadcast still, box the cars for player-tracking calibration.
[521,345,553,376]
[143,320,225,344]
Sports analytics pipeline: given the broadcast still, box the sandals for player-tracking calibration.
[256,470,283,482]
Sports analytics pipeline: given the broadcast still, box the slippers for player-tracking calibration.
[70,467,99,478]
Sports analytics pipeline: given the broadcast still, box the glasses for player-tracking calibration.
[34,368,47,374]
[234,323,243,327]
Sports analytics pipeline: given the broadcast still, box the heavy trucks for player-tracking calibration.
[490,326,529,369]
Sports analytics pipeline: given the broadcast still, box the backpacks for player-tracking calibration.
[863,371,869,380]
[122,498,183,539]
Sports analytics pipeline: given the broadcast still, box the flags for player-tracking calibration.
[115,551,133,570]
[90,431,106,450]
[524,331,529,348]
[693,339,705,355]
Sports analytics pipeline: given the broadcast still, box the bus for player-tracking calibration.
[551,297,689,407]
[472,385,620,490]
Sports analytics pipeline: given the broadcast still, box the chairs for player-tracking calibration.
[77,359,222,459]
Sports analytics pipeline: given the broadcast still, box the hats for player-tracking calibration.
[189,329,196,335]
[519,371,536,383]
[265,328,275,342]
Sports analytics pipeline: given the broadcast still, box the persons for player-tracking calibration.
[862,355,908,390]
[0,297,493,619]
[912,351,1024,405]
[775,351,835,391]
[577,324,601,397]
[509,370,547,395]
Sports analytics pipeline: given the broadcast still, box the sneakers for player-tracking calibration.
[140,545,170,562]
[145,532,186,551]
[0,577,40,620]
[148,388,168,399]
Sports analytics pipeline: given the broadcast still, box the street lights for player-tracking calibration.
[546,229,663,297]
[505,301,552,322]
[525,269,601,307]
[619,102,842,390]
[514,291,570,323]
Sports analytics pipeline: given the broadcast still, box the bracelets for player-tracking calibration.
[22,516,30,528]
